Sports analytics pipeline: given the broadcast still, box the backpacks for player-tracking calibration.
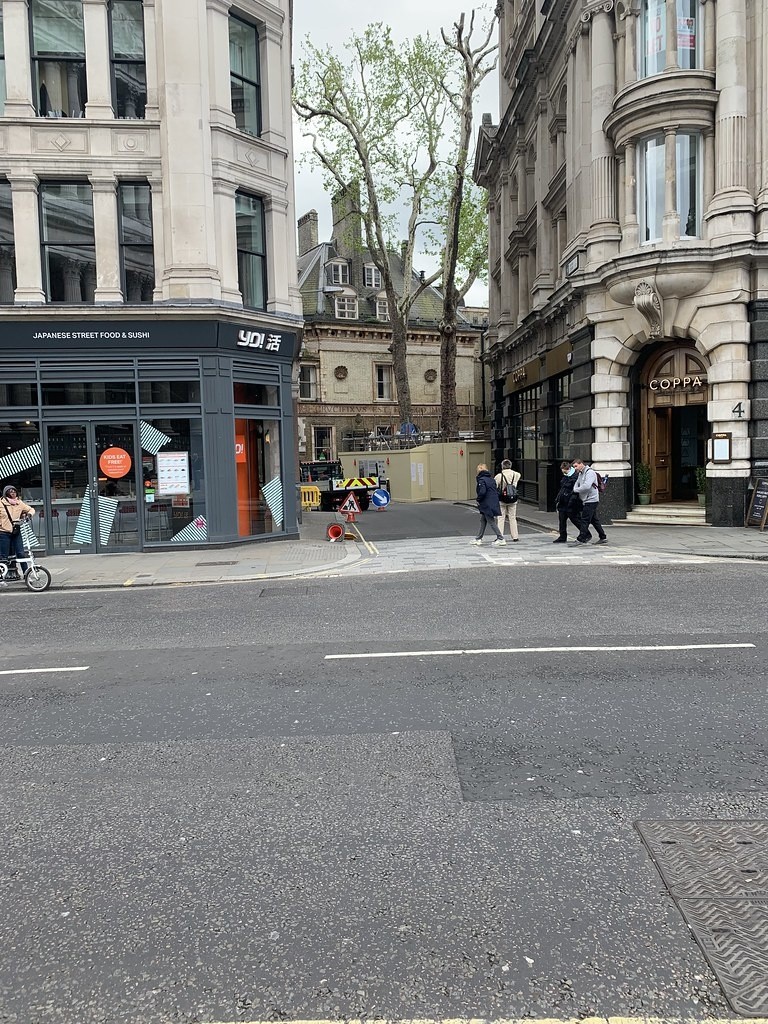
[585,468,606,492]
[500,471,519,504]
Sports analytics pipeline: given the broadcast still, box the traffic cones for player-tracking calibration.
[327,523,345,542]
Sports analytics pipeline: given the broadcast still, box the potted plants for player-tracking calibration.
[695,467,706,506]
[634,462,652,505]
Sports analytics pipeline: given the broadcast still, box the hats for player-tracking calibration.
[3,486,17,497]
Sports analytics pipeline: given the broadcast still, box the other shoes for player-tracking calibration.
[553,538,566,543]
[595,539,607,544]
[513,539,518,541]
[569,540,582,547]
[584,537,591,543]
[0,581,8,587]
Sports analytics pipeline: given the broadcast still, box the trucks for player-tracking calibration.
[298,459,380,512]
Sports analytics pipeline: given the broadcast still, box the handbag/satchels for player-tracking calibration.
[12,524,20,536]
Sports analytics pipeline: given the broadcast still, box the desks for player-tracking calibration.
[21,497,169,547]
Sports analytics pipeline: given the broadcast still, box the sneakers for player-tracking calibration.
[470,539,482,545]
[491,538,506,546]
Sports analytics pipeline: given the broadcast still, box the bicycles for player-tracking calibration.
[0,513,52,592]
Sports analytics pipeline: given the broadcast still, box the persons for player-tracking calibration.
[567,459,608,547]
[553,462,592,543]
[493,459,521,542]
[470,463,506,546]
[0,486,35,578]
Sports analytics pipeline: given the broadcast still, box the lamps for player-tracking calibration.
[300,287,344,294]
